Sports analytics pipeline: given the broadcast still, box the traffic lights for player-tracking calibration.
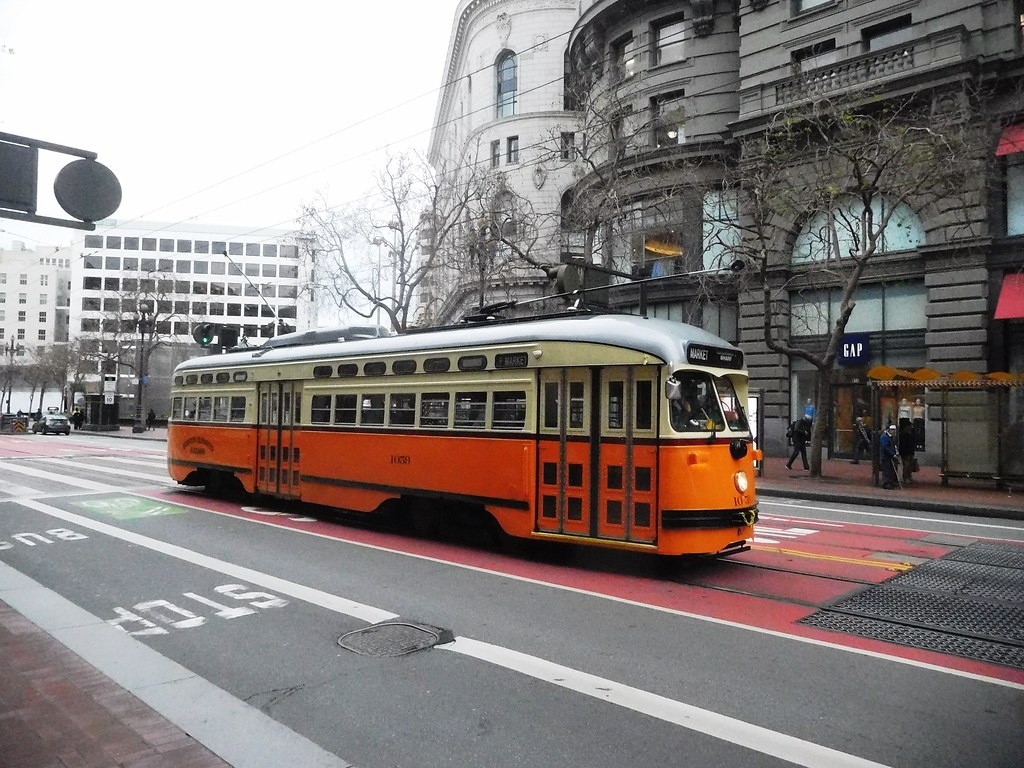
[194,322,214,345]
[548,265,580,300]
[218,326,238,347]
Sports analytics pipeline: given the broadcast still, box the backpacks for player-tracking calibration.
[786,419,800,437]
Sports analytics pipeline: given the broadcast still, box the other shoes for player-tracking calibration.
[882,483,893,490]
[785,465,792,470]
[804,468,810,472]
[850,460,859,464]
[903,479,913,485]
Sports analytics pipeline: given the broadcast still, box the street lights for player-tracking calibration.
[133,302,155,433]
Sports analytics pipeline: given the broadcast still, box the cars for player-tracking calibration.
[32,415,70,435]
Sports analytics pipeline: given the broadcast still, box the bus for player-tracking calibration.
[167,249,765,563]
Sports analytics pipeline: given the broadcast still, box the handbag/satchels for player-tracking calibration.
[912,459,920,473]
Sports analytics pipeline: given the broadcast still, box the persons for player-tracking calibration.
[802,399,817,445]
[71,407,85,430]
[147,409,157,432]
[785,414,812,470]
[312,396,524,426]
[849,410,917,489]
[186,401,202,420]
[897,398,926,451]
[16,408,42,424]
[676,398,702,432]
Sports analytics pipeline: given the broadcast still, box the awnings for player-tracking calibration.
[993,271,1024,322]
[996,123,1024,156]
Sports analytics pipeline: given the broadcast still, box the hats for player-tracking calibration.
[804,414,812,420]
[902,420,912,426]
[884,424,896,436]
